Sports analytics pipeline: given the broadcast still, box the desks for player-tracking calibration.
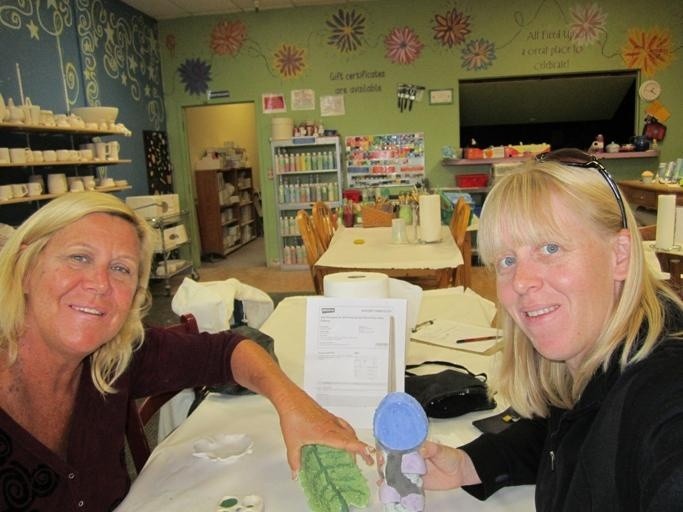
[106,287,540,512]
[618,174,683,214]
[315,225,454,292]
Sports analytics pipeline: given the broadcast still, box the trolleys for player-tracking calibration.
[116,204,213,302]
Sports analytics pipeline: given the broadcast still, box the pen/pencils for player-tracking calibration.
[398,185,427,205]
[456,336,499,343]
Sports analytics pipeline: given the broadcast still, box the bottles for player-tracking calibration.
[283,239,308,264]
[362,187,390,202]
[56,114,116,130]
[279,174,339,203]
[293,118,324,140]
[405,191,411,204]
[641,158,683,183]
[651,139,657,149]
[398,192,405,205]
[280,208,340,235]
[596,134,604,153]
[0,95,55,126]
[275,151,333,172]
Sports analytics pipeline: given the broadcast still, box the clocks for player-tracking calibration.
[639,80,663,101]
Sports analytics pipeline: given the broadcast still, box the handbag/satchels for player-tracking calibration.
[406,369,497,419]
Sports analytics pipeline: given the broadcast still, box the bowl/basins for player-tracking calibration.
[71,107,119,126]
[325,130,335,136]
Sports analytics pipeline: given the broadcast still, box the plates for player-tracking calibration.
[191,433,254,461]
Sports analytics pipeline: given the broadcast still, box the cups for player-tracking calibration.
[101,177,113,186]
[0,173,95,199]
[606,141,620,153]
[0,137,121,163]
[344,213,354,227]
[391,218,408,244]
[399,205,412,225]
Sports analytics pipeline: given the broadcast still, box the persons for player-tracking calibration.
[0,191,376,512]
[376,155,683,512]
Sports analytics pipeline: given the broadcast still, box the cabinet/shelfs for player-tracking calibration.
[140,208,199,294]
[270,138,344,272]
[0,98,132,206]
[431,149,662,196]
[195,166,259,259]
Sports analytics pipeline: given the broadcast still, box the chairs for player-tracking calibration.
[452,196,470,247]
[295,202,336,269]
[125,313,199,474]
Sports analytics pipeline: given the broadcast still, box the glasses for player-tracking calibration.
[534,147,628,229]
